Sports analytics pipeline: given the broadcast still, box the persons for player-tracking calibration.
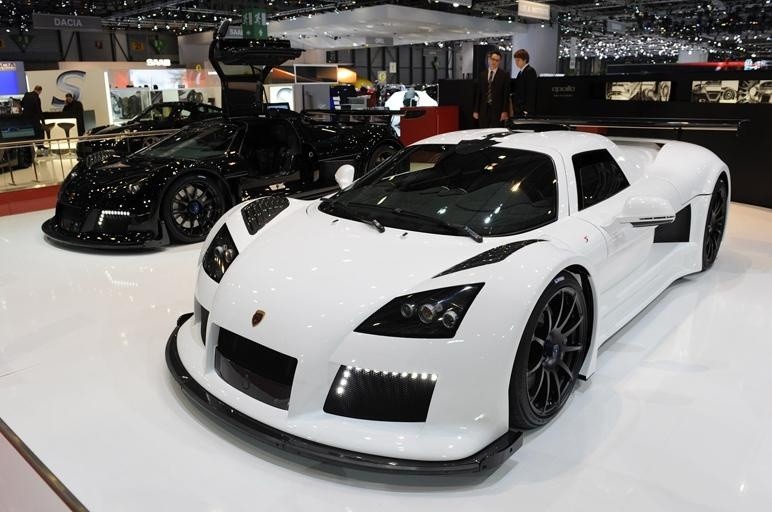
[63,93,85,137]
[473,51,510,128]
[19,85,46,140]
[511,49,540,119]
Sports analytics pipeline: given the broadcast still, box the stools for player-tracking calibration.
[43,123,56,157]
[58,123,75,157]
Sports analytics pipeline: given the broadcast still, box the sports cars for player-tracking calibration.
[166,127,731,476]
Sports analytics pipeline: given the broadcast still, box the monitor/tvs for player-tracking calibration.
[264,102,290,109]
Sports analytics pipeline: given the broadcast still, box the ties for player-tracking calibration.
[487,71,493,107]
